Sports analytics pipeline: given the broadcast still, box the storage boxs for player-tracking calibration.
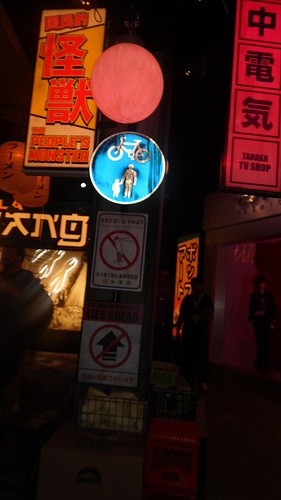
[150,361,175,386]
[36,423,146,500]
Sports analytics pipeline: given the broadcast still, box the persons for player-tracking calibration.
[248,276,276,368]
[0,244,43,416]
[176,278,213,391]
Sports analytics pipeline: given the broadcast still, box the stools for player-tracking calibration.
[143,416,200,500]
